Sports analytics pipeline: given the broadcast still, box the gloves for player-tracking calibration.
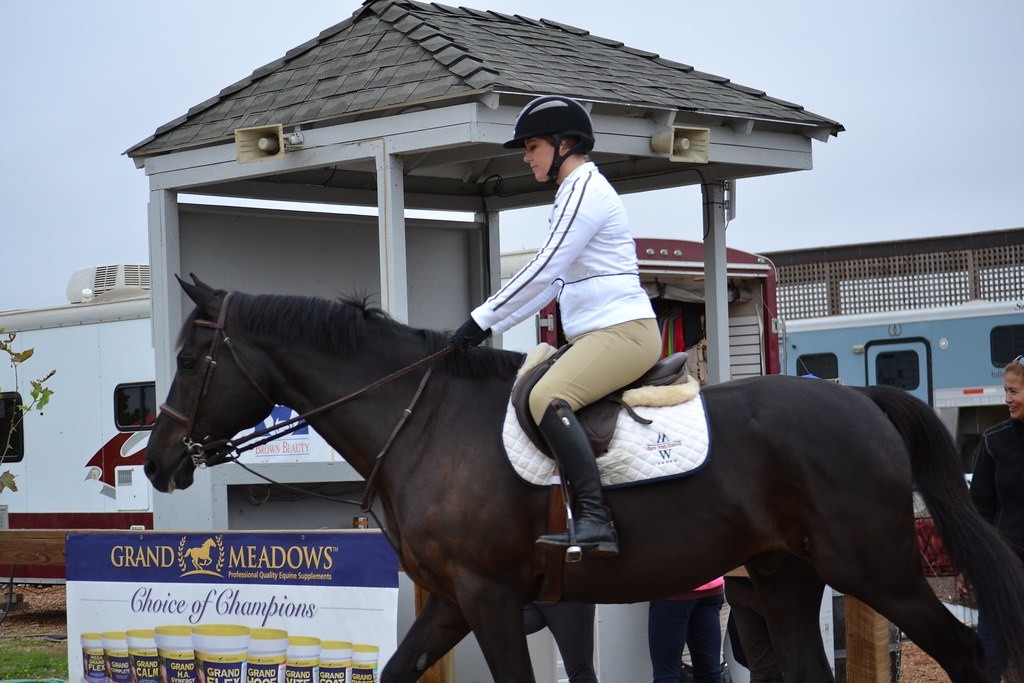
[468,327,492,348]
[449,318,486,353]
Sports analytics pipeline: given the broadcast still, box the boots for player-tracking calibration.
[534,396,621,561]
[718,663,733,683]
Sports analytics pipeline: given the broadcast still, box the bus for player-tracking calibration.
[776,300,1024,577]
[0,238,782,585]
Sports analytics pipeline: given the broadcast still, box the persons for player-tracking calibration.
[524,601,600,683]
[449,96,663,556]
[648,576,728,682]
[953,356,1024,683]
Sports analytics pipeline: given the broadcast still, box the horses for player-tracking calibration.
[143,268,1023,683]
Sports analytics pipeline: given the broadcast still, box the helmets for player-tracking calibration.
[503,94,596,150]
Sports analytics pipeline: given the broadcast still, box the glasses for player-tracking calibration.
[1012,354,1024,371]
